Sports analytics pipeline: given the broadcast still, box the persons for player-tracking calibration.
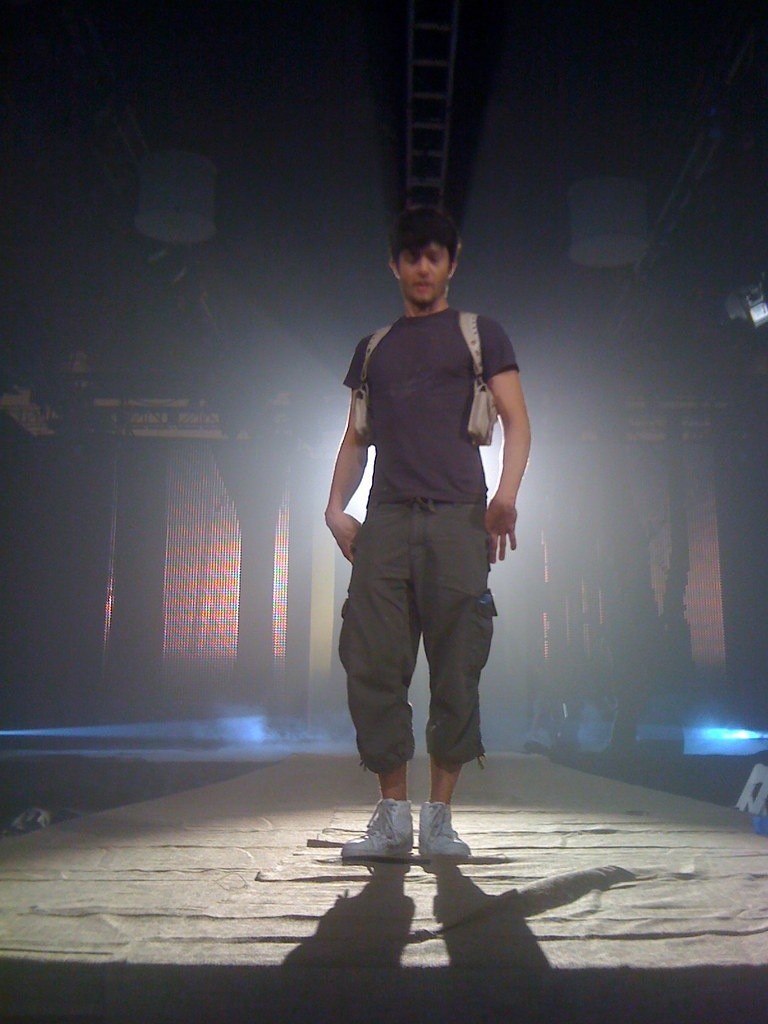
[324,202,532,863]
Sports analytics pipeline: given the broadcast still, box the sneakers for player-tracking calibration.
[420,801,470,857]
[341,798,413,860]
